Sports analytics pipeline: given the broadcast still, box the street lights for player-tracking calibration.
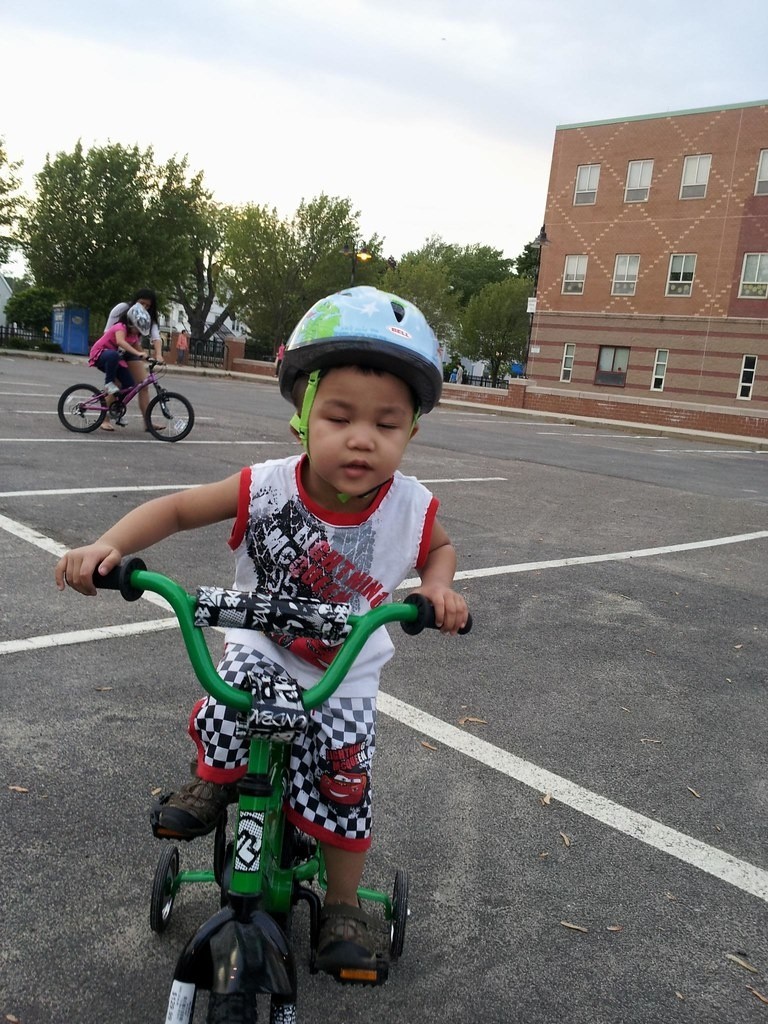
[435,285,454,340]
[338,236,372,289]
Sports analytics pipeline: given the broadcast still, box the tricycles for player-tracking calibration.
[91,555,474,1024]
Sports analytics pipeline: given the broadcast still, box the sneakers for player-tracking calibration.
[158,758,233,837]
[315,901,376,965]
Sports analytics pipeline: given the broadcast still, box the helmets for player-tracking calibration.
[127,302,151,336]
[277,285,443,417]
[453,369,457,372]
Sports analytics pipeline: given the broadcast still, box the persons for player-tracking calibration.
[449,362,464,384]
[55,279,473,979]
[174,329,189,367]
[274,340,285,377]
[86,288,168,433]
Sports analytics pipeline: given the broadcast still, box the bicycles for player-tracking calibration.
[58,355,195,443]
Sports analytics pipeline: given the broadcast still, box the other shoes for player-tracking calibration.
[145,423,166,431]
[104,382,119,394]
[101,421,115,430]
[110,411,128,425]
